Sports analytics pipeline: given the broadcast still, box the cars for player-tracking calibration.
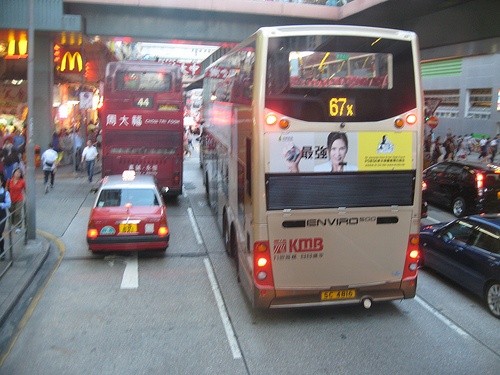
[422,161,500,219]
[419,213,500,320]
[85,171,169,254]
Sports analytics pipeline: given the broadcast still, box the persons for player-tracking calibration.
[0,118,99,183]
[81,139,100,183]
[0,173,12,260]
[425,128,500,163]
[5,167,26,232]
[283,131,359,173]
[183,123,202,158]
[40,142,59,194]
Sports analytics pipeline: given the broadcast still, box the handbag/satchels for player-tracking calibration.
[7,179,10,192]
[45,161,54,166]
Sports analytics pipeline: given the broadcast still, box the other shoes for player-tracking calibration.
[15,228,22,234]
[1,252,7,261]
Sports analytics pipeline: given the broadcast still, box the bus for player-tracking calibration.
[96,61,183,205]
[201,25,424,310]
